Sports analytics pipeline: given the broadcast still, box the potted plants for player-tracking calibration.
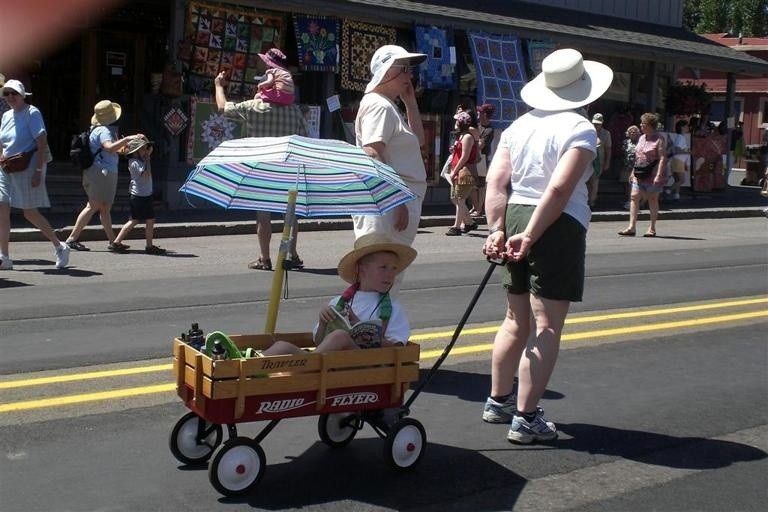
[658,80,715,117]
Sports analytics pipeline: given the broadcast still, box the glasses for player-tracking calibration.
[391,65,412,74]
[3,91,18,97]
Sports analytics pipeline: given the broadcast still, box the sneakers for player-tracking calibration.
[53,243,71,269]
[445,228,462,237]
[247,256,274,272]
[481,395,546,424]
[619,228,636,236]
[286,255,305,270]
[506,416,560,445]
[66,240,91,251]
[469,207,482,217]
[644,228,658,238]
[245,348,266,361]
[0,254,14,270]
[109,240,131,250]
[145,245,166,254]
[462,223,479,234]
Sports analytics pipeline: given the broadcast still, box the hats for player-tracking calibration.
[477,104,496,116]
[257,48,289,71]
[336,232,418,286]
[519,47,614,113]
[90,100,122,126]
[0,79,32,98]
[591,113,604,125]
[364,44,428,94]
[453,113,472,124]
[254,74,268,82]
[125,139,153,155]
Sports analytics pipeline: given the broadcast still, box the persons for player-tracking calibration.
[443,103,694,236]
[350,45,429,300]
[260,233,418,373]
[110,133,160,254]
[216,47,311,271]
[0,73,10,121]
[481,48,617,441]
[65,99,137,251]
[0,79,71,269]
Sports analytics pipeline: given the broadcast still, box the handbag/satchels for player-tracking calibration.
[476,154,487,177]
[633,165,652,180]
[457,162,480,186]
[0,152,32,174]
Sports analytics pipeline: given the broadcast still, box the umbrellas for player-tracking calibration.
[178,134,420,334]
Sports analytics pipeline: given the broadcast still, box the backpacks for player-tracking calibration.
[69,130,95,171]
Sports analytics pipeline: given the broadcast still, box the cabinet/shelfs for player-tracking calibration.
[658,127,730,199]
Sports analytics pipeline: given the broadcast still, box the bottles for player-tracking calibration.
[190,323,204,349]
[192,337,201,351]
[211,339,232,360]
